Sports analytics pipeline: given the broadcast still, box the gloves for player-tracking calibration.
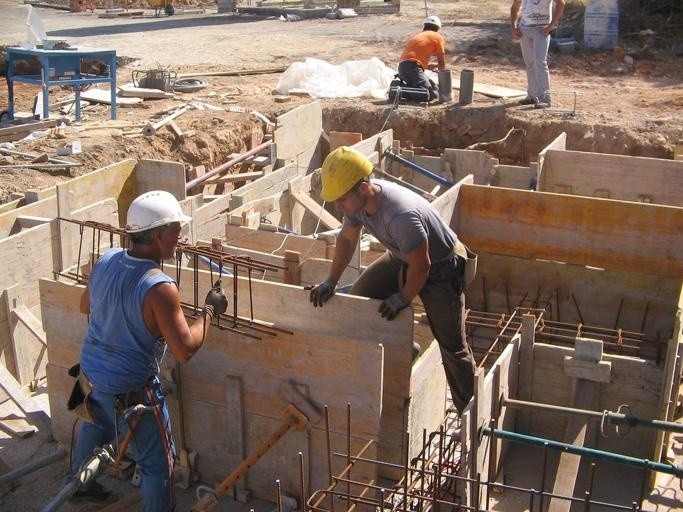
[379,289,409,320]
[304,278,336,308]
[202,279,229,319]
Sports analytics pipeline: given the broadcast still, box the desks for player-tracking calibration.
[5,46,117,123]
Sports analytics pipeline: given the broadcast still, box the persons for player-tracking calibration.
[67,190,229,512]
[302,145,480,445]
[510,0,565,109]
[388,15,446,102]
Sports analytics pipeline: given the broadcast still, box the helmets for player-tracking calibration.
[320,146,373,201]
[126,190,193,233]
[422,16,441,28]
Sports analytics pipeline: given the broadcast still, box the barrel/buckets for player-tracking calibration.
[583,0,619,50]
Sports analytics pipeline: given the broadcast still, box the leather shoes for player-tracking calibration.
[519,97,534,105]
[73,481,119,507]
[535,100,550,108]
